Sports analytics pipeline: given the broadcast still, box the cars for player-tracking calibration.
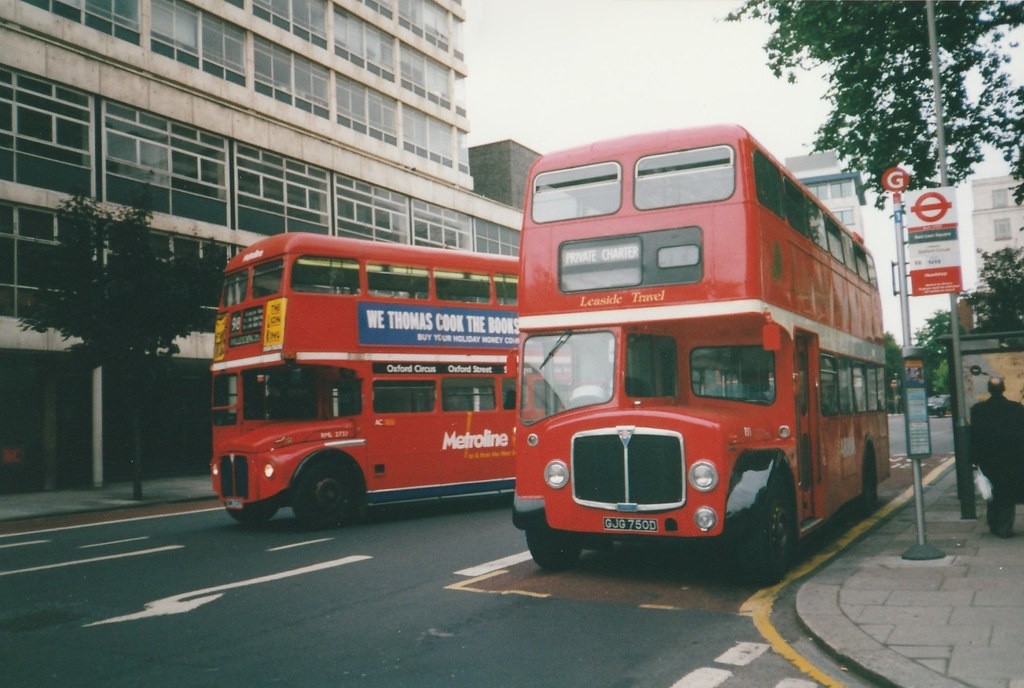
[927,394,953,418]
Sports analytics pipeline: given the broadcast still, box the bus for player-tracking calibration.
[502,123,893,587]
[208,232,613,532]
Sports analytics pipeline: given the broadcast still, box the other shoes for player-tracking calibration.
[991,529,1013,539]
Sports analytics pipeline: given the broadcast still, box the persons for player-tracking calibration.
[260,378,288,418]
[965,370,1024,538]
